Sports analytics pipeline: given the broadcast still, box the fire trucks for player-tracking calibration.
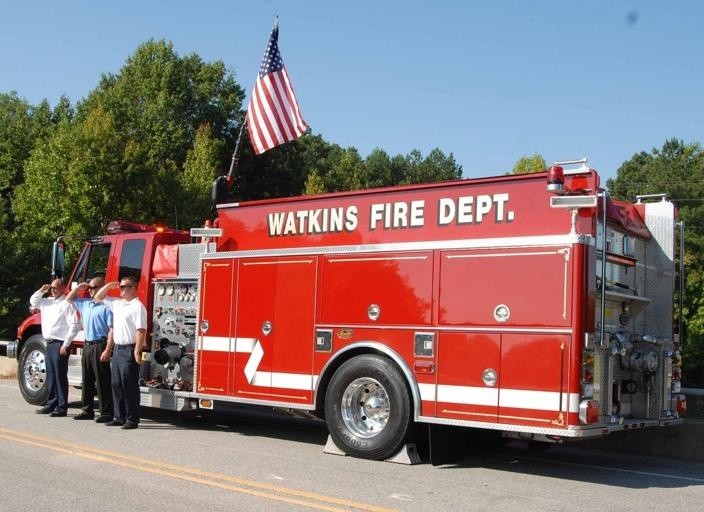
[3,154,686,467]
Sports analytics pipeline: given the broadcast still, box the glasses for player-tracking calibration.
[50,286,60,290]
[87,286,100,290]
[118,285,134,289]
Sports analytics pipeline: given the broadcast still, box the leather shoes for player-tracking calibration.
[34,407,52,413]
[121,423,137,429]
[104,421,123,426]
[95,416,114,423]
[73,412,94,420]
[49,411,66,416]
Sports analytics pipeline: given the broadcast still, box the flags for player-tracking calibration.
[243,21,309,157]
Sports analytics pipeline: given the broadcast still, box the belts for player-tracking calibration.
[84,339,102,345]
[46,340,60,344]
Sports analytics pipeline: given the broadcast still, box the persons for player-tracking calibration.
[93,276,149,430]
[65,277,114,423]
[29,277,79,418]
